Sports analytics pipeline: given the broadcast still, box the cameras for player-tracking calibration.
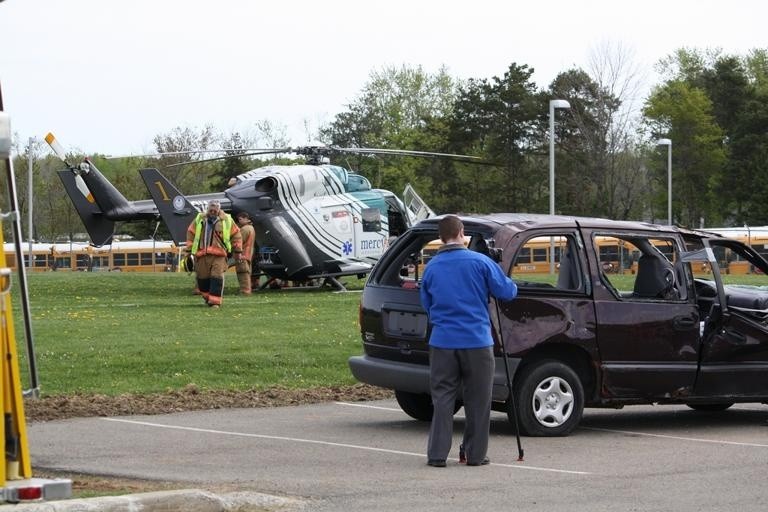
[474,234,503,262]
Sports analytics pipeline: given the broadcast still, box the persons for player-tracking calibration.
[419,214,519,466]
[233,213,256,294]
[186,199,242,308]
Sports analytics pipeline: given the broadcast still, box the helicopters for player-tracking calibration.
[43,130,488,295]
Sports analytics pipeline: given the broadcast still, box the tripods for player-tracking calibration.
[457,295,539,460]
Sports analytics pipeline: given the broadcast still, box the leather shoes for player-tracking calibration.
[466,456,488,465]
[428,459,446,466]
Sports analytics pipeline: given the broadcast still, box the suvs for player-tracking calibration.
[348,210,768,435]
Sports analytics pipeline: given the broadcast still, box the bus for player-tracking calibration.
[3,241,235,273]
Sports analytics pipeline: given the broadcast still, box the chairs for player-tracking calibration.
[633,255,677,300]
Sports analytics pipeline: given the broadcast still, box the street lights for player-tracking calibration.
[657,137,674,226]
[547,99,571,216]
[27,136,37,268]
